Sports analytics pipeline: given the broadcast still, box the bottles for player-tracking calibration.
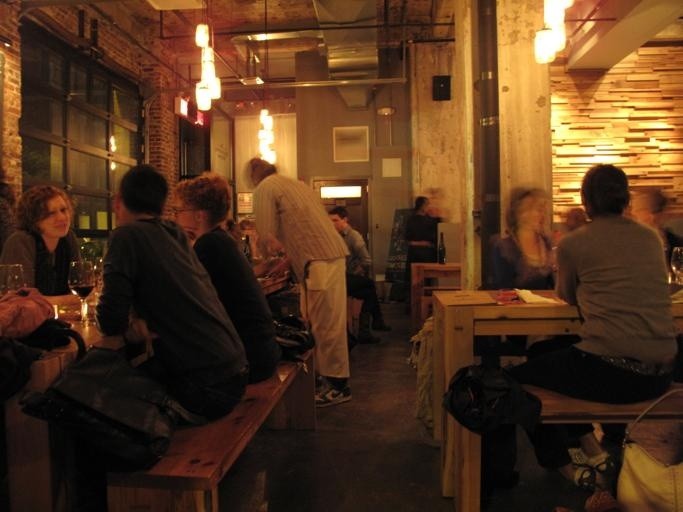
[438,232,446,264]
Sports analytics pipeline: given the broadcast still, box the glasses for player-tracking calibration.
[171,207,194,214]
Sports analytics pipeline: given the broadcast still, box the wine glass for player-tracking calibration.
[670,247,682,284]
[68,260,95,326]
[0,264,26,298]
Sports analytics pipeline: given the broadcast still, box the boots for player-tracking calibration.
[372,303,392,331]
[358,312,381,344]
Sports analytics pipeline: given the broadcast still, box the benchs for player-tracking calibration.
[101,352,316,512]
[452,382,683,512]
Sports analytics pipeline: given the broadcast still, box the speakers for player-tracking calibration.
[432,75,450,101]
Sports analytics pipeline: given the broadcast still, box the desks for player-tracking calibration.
[433,289,683,500]
[1,319,135,512]
[411,262,460,338]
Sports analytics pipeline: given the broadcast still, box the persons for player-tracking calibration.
[525,164,679,489]
[93,164,250,429]
[0,183,83,296]
[244,157,353,409]
[228,206,393,345]
[481,182,682,353]
[173,173,281,387]
[406,197,442,291]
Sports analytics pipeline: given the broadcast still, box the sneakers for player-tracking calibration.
[560,465,603,487]
[568,448,614,473]
[315,375,324,391]
[314,383,352,408]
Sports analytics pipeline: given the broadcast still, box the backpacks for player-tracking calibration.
[271,312,314,375]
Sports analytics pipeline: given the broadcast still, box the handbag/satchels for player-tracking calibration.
[44,346,208,473]
[442,362,542,435]
[616,388,683,512]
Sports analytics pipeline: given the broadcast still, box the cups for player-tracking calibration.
[93,257,104,295]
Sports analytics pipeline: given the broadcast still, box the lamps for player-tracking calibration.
[534,1,573,68]
[258,64,279,169]
[192,0,224,115]
